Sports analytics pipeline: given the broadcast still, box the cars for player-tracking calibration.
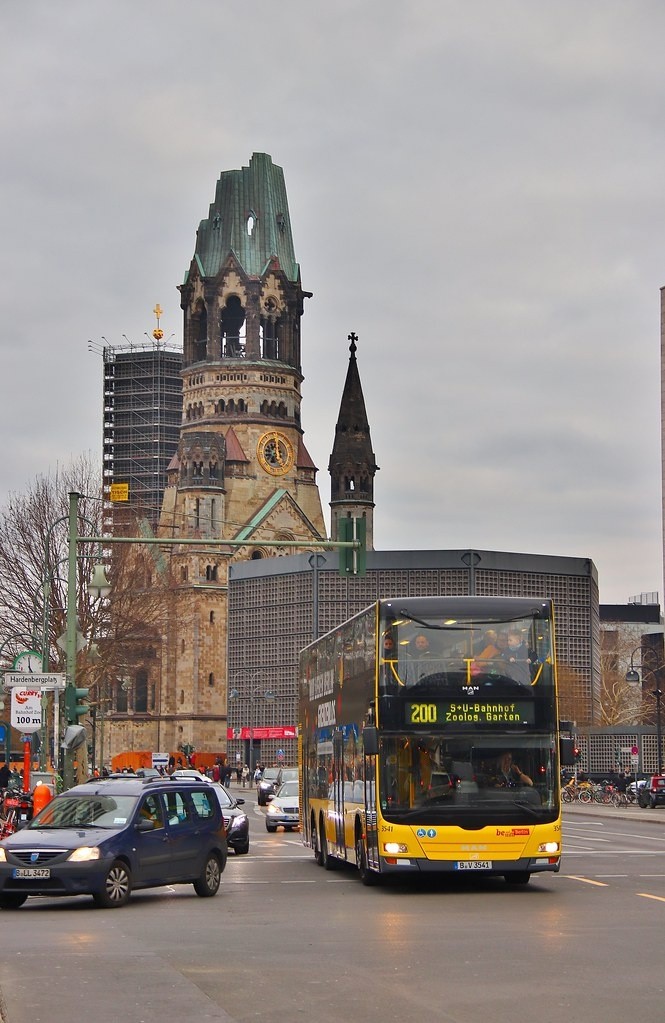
[171,769,212,783]
[272,768,299,794]
[163,781,250,854]
[638,777,665,809]
[266,781,301,832]
[135,769,162,778]
[256,768,281,806]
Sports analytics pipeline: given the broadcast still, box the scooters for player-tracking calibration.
[0,780,43,824]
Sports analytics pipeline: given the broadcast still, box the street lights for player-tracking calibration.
[33,512,112,775]
[624,645,663,777]
[229,671,275,789]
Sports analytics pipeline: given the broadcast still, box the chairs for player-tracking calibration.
[387,651,448,686]
[83,801,105,826]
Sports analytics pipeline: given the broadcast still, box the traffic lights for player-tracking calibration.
[66,686,89,722]
[576,749,582,762]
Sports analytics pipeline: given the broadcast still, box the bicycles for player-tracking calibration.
[562,783,632,809]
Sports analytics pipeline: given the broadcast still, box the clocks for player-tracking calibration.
[256,431,295,476]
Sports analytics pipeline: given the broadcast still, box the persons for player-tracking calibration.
[128,801,160,828]
[0,763,19,787]
[94,755,291,790]
[560,765,657,795]
[383,629,542,685]
[484,752,536,788]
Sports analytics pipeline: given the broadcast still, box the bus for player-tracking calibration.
[300,597,579,887]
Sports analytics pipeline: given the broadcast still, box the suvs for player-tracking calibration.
[0,775,228,910]
